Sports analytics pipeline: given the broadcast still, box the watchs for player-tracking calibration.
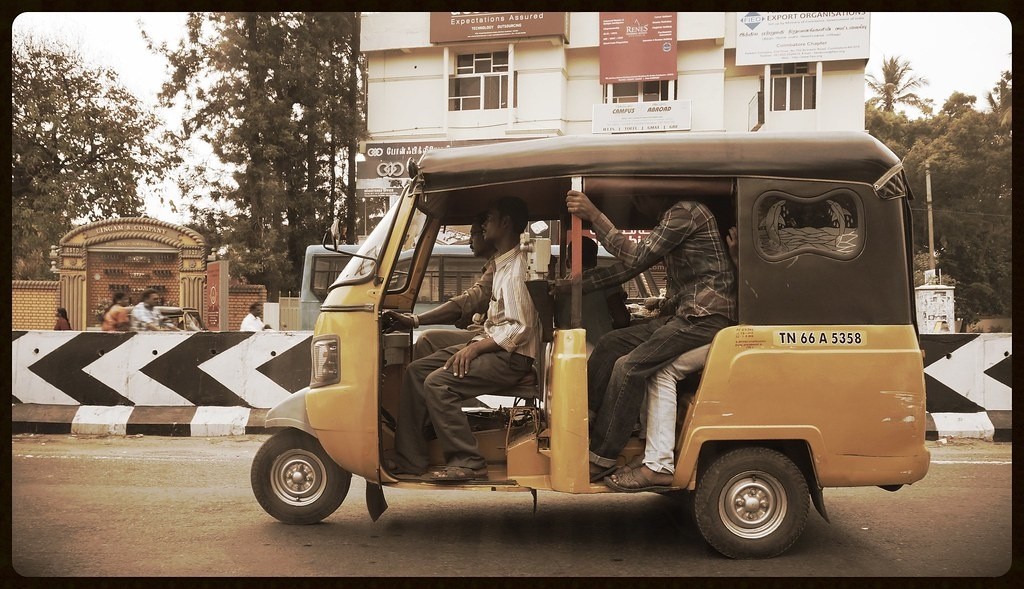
[410,313,419,329]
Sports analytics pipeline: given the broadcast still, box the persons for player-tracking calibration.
[95,289,174,331]
[548,190,739,494]
[53,308,73,331]
[240,304,272,331]
[381,196,541,481]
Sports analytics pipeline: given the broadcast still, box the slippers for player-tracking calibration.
[611,454,645,473]
[383,447,425,474]
[588,465,615,482]
[603,474,673,492]
[431,463,490,481]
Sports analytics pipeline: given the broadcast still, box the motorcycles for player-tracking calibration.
[251,131,938,559]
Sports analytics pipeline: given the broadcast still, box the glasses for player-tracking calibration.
[471,230,481,237]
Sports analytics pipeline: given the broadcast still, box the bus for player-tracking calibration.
[297,239,663,330]
